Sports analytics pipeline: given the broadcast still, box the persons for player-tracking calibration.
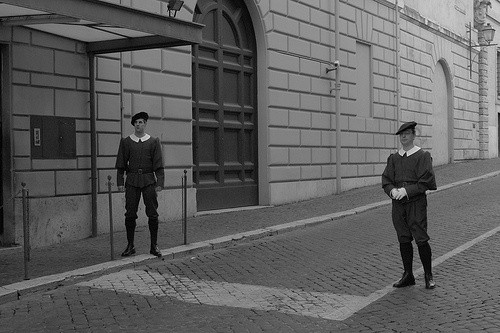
[382,121,436,288]
[115,112,165,256]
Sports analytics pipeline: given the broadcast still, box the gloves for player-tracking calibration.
[391,188,408,200]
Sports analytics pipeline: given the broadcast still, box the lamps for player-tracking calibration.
[167,0,184,18]
[467,22,498,79]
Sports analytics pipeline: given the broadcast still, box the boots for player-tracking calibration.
[148,221,162,257]
[393,243,415,287]
[122,224,136,256]
[418,244,435,289]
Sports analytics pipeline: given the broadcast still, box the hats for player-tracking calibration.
[395,121,417,136]
[131,112,148,127]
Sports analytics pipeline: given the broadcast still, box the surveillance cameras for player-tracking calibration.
[334,61,339,67]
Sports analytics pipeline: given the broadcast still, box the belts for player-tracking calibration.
[127,168,153,175]
